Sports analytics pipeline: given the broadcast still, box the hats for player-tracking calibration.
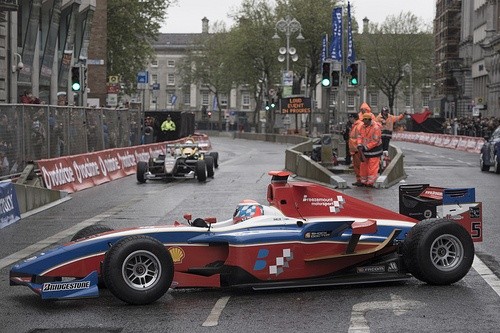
[362,113,373,119]
[361,103,370,111]
[383,105,389,110]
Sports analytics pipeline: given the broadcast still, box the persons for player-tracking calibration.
[344,103,405,186]
[0,106,144,176]
[161,115,177,131]
[233,200,265,224]
[182,148,194,156]
[441,117,499,139]
[21,90,40,104]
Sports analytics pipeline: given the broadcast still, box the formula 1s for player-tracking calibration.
[10,171,482,306]
[137,142,218,182]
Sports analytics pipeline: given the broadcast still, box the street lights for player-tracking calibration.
[272,16,305,72]
[401,63,412,114]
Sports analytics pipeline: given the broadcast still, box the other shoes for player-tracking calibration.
[357,182,373,188]
[352,181,358,185]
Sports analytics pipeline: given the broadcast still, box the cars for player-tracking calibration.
[480,125,500,174]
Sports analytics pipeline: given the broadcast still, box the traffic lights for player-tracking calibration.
[266,101,269,111]
[72,66,80,92]
[350,63,360,85]
[322,62,330,87]
[209,111,211,118]
[271,98,275,108]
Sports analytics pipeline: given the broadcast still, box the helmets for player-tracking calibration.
[233,199,264,224]
